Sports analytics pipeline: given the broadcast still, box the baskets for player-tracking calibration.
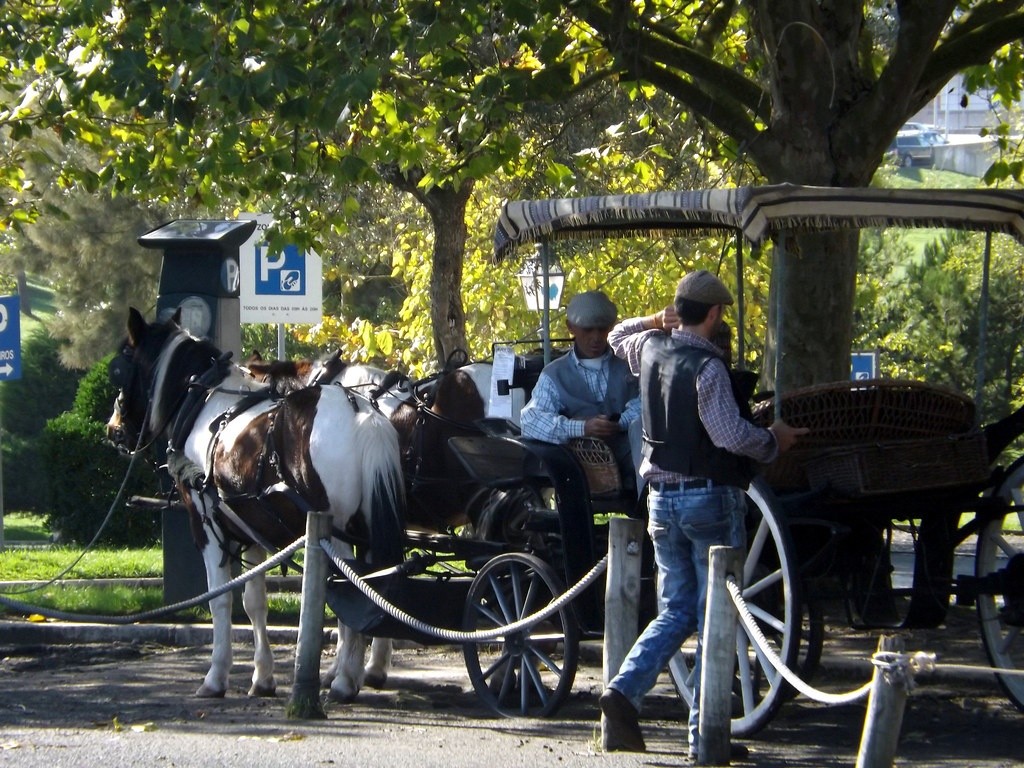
[758,380,988,494]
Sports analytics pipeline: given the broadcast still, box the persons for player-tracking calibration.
[519,290,650,514]
[599,270,813,759]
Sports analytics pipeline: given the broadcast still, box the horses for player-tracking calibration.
[253,356,537,690]
[108,304,402,702]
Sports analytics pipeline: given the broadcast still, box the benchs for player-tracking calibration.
[476,417,641,527]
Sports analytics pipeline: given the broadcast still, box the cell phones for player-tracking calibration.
[608,413,621,423]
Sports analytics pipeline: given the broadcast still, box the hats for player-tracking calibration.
[566,290,618,328]
[675,269,735,305]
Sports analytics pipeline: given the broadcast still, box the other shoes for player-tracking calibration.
[689,742,750,760]
[599,688,646,752]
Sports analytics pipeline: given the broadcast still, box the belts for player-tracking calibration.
[651,480,718,491]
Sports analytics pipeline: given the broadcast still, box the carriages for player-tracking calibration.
[110,182,1024,740]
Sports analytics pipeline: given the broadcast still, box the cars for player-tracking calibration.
[885,129,951,169]
[895,122,936,133]
